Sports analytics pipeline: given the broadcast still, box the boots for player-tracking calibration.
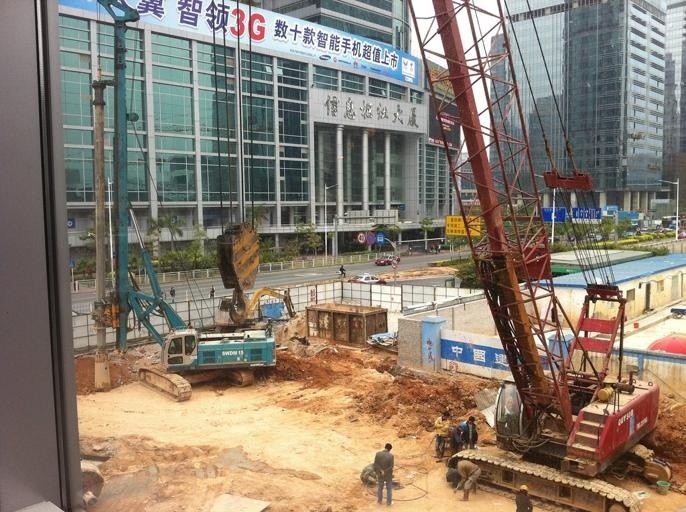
[459,488,470,501]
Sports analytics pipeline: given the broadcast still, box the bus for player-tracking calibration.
[661,216,680,228]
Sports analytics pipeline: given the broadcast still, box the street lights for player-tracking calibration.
[324,183,339,256]
[657,177,679,243]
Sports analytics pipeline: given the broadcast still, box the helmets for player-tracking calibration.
[519,484,529,492]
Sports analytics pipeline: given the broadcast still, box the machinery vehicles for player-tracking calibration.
[406,0,674,511]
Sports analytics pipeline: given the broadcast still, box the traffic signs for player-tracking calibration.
[358,233,365,244]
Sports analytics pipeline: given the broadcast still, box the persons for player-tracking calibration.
[373,441,395,505]
[464,415,478,450]
[515,484,534,512]
[432,409,451,462]
[339,265,346,278]
[447,457,481,502]
[450,421,468,452]
[169,286,176,303]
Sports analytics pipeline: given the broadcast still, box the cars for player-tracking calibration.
[348,272,386,285]
[375,254,400,265]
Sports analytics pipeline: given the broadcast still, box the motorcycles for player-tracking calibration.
[336,270,346,278]
